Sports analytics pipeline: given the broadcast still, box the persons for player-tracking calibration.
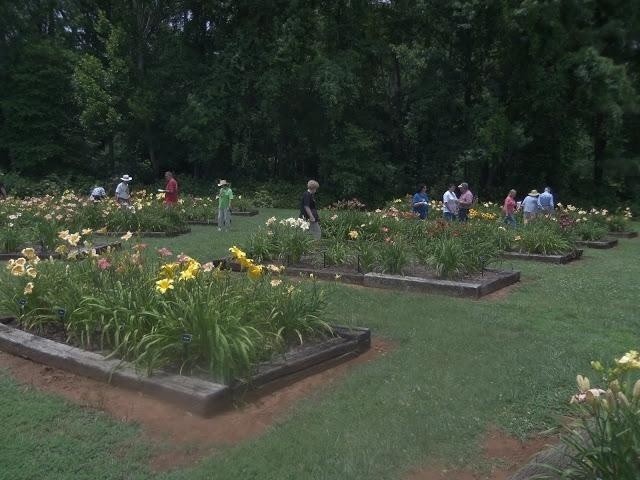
[458,182,474,220]
[216,178,234,232]
[91,187,107,203]
[162,172,178,206]
[411,182,432,220]
[300,178,324,242]
[501,188,520,229]
[520,190,546,224]
[538,187,554,212]
[442,184,461,222]
[115,175,134,205]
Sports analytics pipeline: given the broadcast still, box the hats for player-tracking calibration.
[119,174,133,182]
[457,182,469,189]
[527,189,540,197]
[217,180,230,187]
